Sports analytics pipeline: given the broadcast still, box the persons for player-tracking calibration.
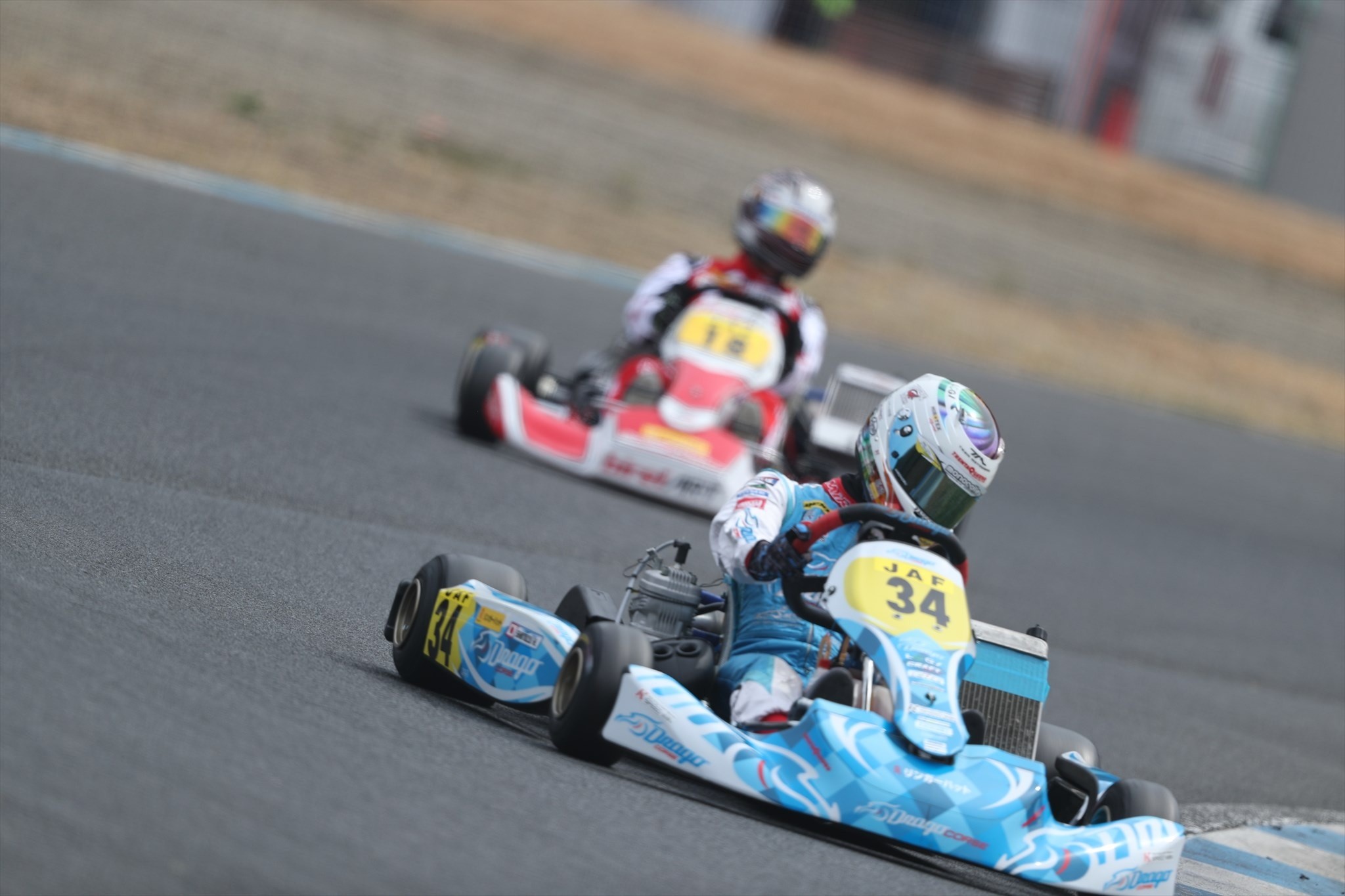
[710,372,1005,745]
[572,170,838,442]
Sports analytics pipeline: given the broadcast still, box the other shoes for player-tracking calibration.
[787,668,854,721]
[958,709,986,745]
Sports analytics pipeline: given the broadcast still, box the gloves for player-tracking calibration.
[746,522,813,582]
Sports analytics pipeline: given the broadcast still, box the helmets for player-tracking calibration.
[855,373,1006,550]
[735,171,837,277]
